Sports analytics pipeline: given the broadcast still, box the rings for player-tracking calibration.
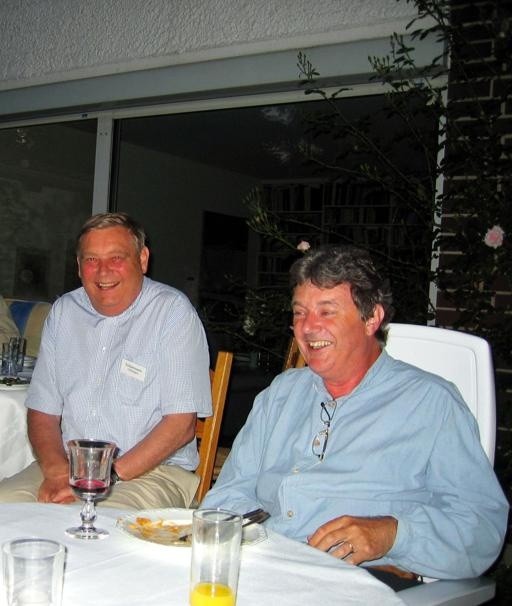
[346,542,355,550]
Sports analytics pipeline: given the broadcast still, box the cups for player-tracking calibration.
[2,536,65,605]
[0,336,27,374]
[188,512,244,606]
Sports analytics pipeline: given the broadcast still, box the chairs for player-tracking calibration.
[193,352,233,503]
[0,297,54,358]
[274,323,498,606]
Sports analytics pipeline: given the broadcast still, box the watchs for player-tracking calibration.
[111,469,122,484]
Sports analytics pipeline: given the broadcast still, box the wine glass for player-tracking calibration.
[66,438,117,541]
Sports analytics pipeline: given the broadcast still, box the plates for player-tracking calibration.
[120,505,269,550]
[0,354,38,391]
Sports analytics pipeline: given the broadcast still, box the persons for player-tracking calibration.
[201,248,510,592]
[0,213,213,511]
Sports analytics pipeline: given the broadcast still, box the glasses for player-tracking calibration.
[308,398,336,460]
